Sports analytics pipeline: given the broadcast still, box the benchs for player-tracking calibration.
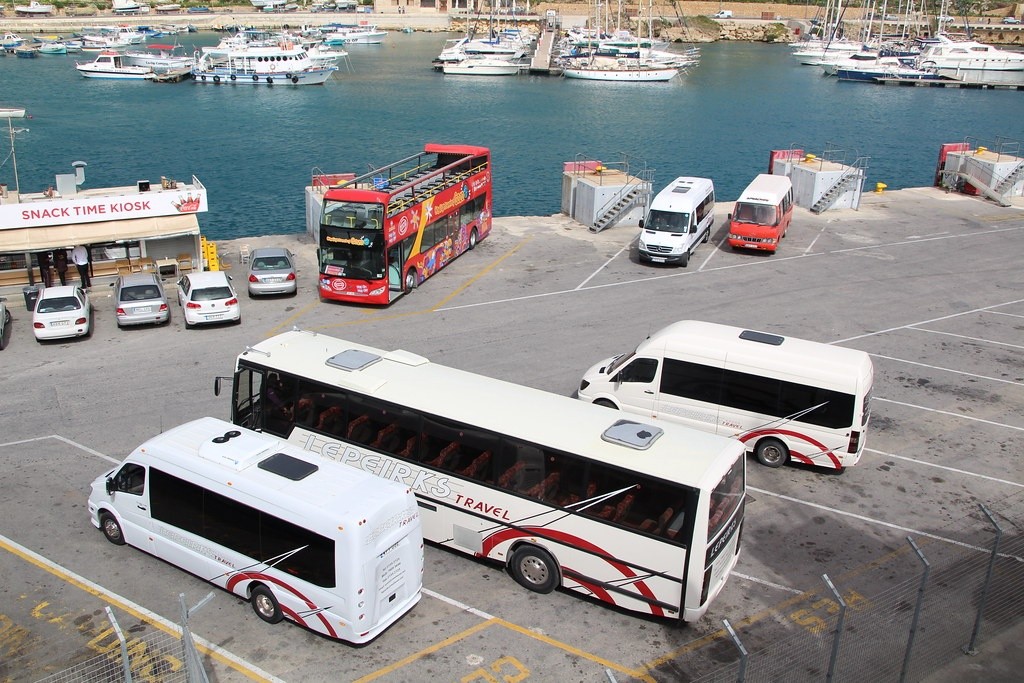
[290,398,742,541]
[39,304,77,312]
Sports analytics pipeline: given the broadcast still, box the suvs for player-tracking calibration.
[1003,17,1020,24]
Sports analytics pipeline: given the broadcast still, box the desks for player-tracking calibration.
[156,259,180,278]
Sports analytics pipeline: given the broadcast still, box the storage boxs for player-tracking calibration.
[201,235,219,271]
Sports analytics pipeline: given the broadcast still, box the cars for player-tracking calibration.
[110,274,171,328]
[868,12,881,20]
[31,286,89,342]
[244,248,297,297]
[177,271,242,329]
[0,294,11,352]
[884,14,899,21]
[935,14,955,22]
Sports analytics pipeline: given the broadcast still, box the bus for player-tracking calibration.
[215,327,756,623]
[317,143,492,304]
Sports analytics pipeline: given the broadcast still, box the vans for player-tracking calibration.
[87,416,424,644]
[727,174,794,255]
[638,176,715,267]
[715,10,732,19]
[578,318,874,469]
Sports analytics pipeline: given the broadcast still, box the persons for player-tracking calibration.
[71,245,91,288]
[398,6,401,14]
[402,6,405,14]
[53,250,67,286]
[267,373,287,413]
[41,257,52,288]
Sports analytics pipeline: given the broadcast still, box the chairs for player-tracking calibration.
[115,259,131,271]
[139,256,156,273]
[126,291,136,300]
[277,261,286,267]
[257,262,265,267]
[177,256,193,274]
[145,290,154,299]
[331,163,486,229]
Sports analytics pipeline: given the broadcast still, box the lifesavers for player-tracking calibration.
[214,76,220,82]
[202,76,206,80]
[190,74,196,80]
[286,73,291,78]
[253,75,258,81]
[231,75,236,80]
[267,77,272,83]
[292,76,298,83]
[270,64,275,70]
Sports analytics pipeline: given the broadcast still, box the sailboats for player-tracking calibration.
[432,0,700,82]
[786,0,1024,81]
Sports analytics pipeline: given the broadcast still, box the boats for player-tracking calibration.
[75,0,413,86]
[0,0,215,58]
